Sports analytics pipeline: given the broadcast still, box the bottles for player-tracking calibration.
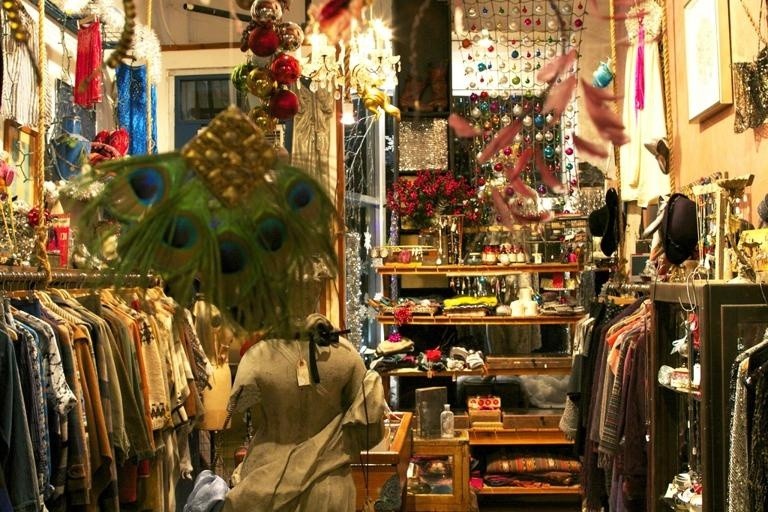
[440,404,455,439]
[690,487,703,512]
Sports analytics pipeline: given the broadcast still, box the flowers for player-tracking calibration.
[383,169,484,228]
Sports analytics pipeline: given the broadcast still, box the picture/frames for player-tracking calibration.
[682,0,734,125]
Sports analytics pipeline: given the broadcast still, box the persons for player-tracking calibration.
[226,265,387,511]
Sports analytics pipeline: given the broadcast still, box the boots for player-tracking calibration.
[644,139,669,174]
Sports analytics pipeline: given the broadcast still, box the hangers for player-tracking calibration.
[0,269,172,342]
[590,283,651,334]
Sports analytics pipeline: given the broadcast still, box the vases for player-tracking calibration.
[418,229,440,266]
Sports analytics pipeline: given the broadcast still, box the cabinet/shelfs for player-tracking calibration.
[375,262,589,498]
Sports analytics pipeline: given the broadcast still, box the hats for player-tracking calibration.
[662,192,699,263]
[589,188,626,256]
[49,139,90,180]
